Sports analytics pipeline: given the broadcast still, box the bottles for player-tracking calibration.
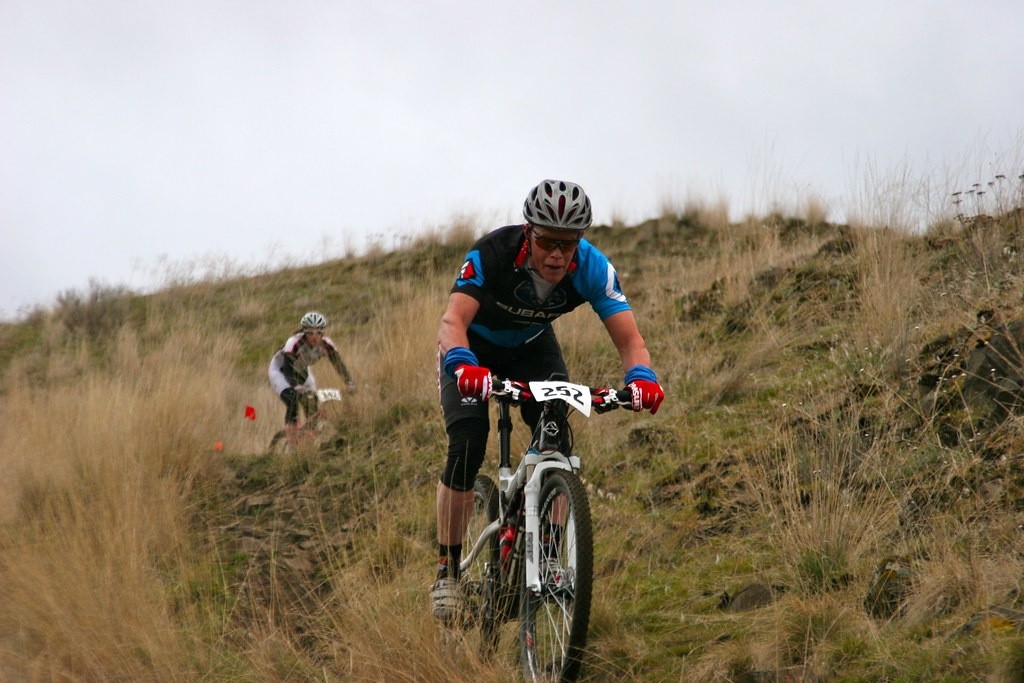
[499,518,516,571]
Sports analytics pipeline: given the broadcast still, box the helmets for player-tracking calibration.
[523,178,593,230]
[301,312,326,328]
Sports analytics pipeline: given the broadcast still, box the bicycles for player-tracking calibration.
[456,377,634,683]
[267,388,354,458]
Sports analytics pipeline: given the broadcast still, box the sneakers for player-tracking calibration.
[539,554,568,583]
[429,575,469,622]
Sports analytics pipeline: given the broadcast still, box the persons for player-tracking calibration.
[428,180,666,630]
[268,312,357,440]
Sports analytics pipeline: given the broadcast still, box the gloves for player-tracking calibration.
[454,363,493,402]
[625,380,665,416]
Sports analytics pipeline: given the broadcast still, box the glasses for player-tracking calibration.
[303,329,325,335]
[528,227,580,251]
